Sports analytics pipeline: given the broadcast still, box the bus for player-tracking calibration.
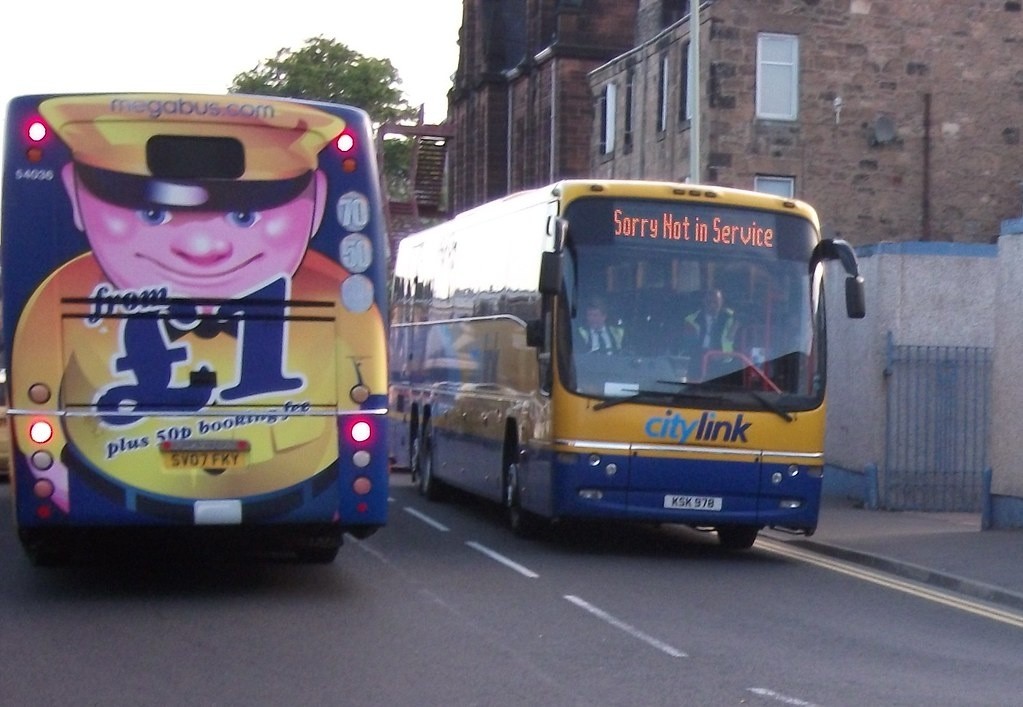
[0,86,395,570]
[385,176,867,554]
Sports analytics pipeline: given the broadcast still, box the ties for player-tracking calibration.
[596,330,606,356]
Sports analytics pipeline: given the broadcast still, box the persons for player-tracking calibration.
[573,296,626,357]
[682,286,739,367]
